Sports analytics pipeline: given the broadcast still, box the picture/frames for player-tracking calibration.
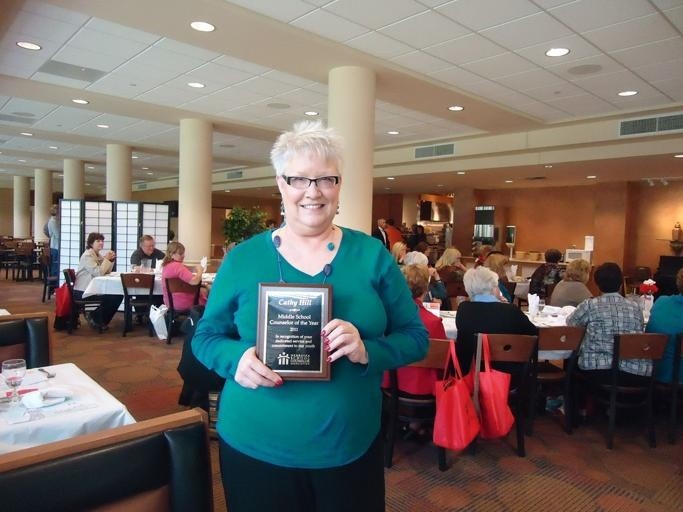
[253,282,335,382]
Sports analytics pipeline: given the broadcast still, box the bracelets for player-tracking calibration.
[435,280,442,282]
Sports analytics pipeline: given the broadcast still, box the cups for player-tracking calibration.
[140,258,151,272]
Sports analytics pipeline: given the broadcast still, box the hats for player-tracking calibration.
[403,250,429,265]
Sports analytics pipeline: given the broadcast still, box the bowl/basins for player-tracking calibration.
[514,251,545,261]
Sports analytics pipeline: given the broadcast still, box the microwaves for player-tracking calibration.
[565,248,592,264]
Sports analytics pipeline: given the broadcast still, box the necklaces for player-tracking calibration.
[273,221,336,285]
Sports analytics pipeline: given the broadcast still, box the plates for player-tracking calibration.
[21,390,63,406]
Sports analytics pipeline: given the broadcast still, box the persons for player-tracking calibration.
[563,261,653,428]
[375,218,438,263]
[130,234,165,327]
[484,254,514,303]
[190,118,430,512]
[435,248,468,297]
[528,248,563,305]
[644,270,680,385]
[452,266,539,389]
[72,233,124,332]
[406,250,446,307]
[549,258,594,308]
[474,244,492,269]
[42,203,60,295]
[158,241,212,313]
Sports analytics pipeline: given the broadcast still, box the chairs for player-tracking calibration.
[1,308,56,379]
[0,398,215,512]
[0,226,216,345]
[378,243,682,473]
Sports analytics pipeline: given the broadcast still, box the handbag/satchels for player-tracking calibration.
[54,282,73,317]
[432,333,515,450]
[148,304,169,340]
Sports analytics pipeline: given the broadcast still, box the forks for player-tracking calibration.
[37,367,55,379]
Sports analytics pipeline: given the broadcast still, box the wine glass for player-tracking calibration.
[527,300,545,323]
[0,359,26,407]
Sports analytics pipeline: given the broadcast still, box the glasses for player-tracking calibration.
[281,175,339,189]
